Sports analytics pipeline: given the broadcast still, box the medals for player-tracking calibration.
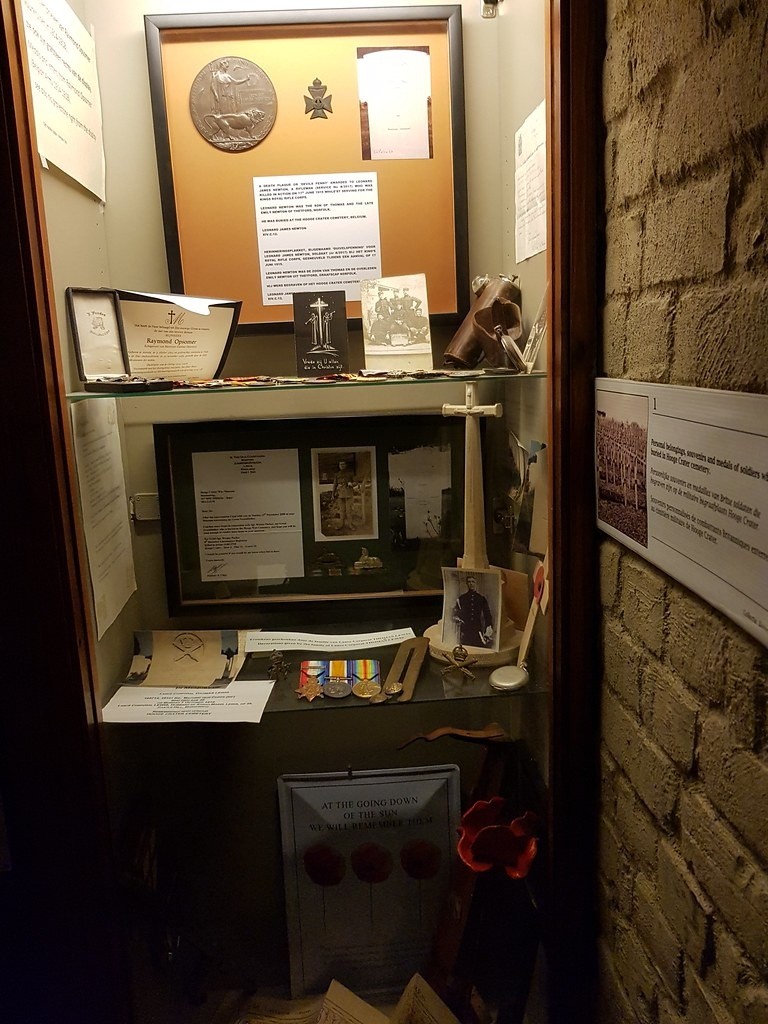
[294,677,382,702]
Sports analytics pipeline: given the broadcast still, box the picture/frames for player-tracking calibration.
[152,412,493,614]
[144,4,472,335]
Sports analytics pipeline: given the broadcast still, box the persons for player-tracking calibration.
[453,576,493,646]
[332,459,360,530]
